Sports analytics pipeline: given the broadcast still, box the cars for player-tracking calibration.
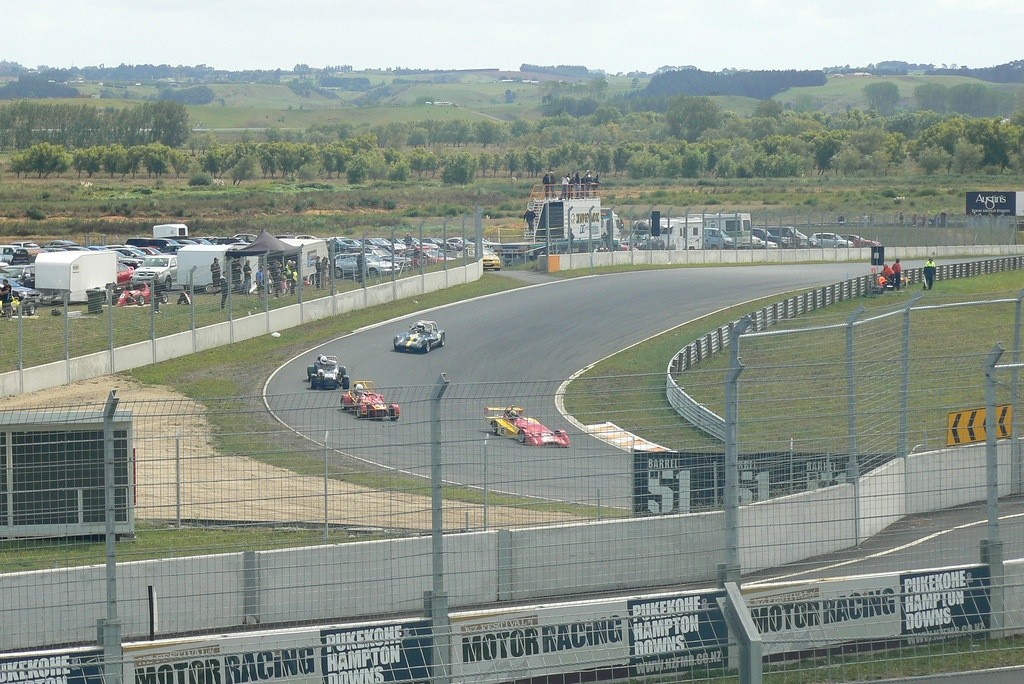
[0,225,885,317]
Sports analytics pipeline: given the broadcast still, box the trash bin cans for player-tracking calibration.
[86,289,106,314]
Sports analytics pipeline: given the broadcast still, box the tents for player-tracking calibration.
[226,228,304,305]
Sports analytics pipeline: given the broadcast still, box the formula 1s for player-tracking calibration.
[308,355,350,390]
[1,296,35,316]
[341,380,401,422]
[482,405,570,447]
[110,280,169,306]
[393,320,446,354]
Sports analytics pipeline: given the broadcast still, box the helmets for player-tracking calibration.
[356,383,365,393]
[509,410,517,418]
[321,355,327,366]
[416,323,424,330]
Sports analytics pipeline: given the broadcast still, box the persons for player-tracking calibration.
[621,243,639,251]
[940,211,947,228]
[509,410,517,420]
[0,279,12,322]
[559,170,602,200]
[357,251,367,284]
[316,255,328,290]
[924,258,936,290]
[543,171,557,199]
[417,322,427,332]
[355,384,366,398]
[405,233,412,248]
[837,215,844,228]
[892,258,901,290]
[211,257,221,296]
[899,212,927,228]
[233,257,296,303]
[878,265,895,294]
[861,213,871,227]
[320,356,328,365]
[220,271,229,310]
[154,271,162,314]
[512,176,518,182]
[524,208,537,231]
[567,228,574,254]
